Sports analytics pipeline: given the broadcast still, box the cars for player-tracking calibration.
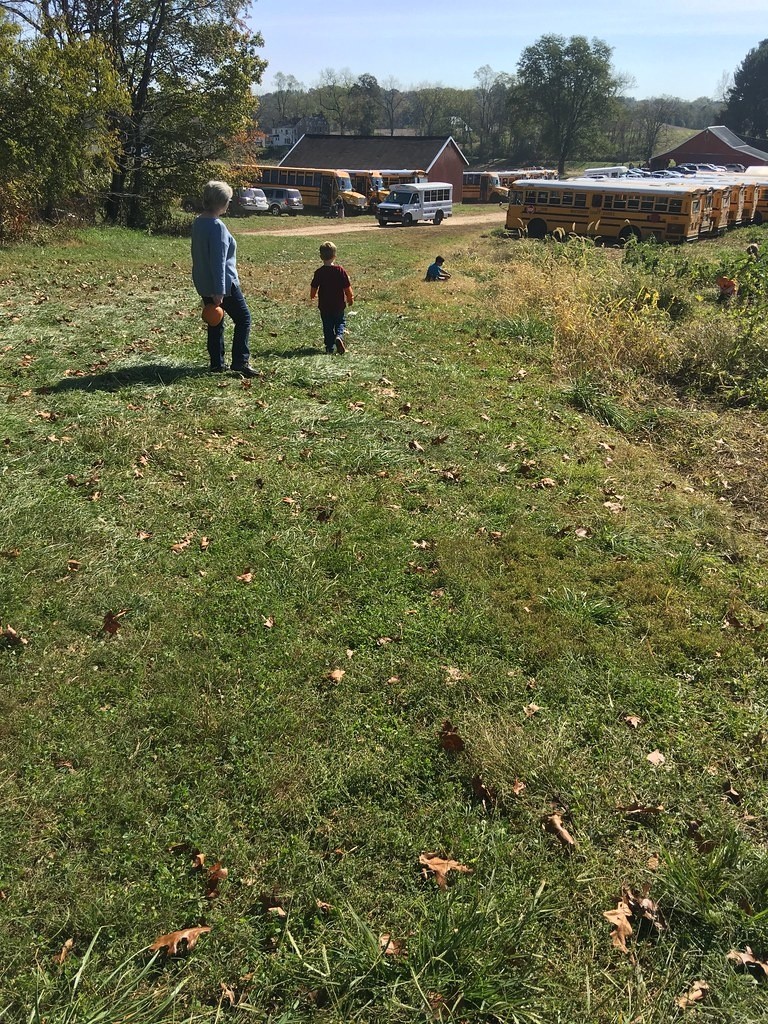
[233,187,304,217]
[566,162,746,179]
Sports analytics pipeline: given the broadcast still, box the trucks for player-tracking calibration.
[375,182,453,227]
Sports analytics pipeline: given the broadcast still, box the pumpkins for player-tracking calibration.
[716,276,737,296]
[201,303,223,326]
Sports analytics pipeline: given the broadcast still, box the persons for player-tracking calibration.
[335,195,344,219]
[425,256,451,281]
[191,180,263,378]
[736,245,758,310]
[716,259,736,310]
[310,242,354,355]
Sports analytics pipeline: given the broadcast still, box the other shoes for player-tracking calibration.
[335,337,345,354]
[231,362,260,378]
[325,345,334,353]
[208,364,230,375]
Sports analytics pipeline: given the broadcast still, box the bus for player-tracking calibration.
[242,165,429,216]
[499,166,768,249]
[462,166,559,203]
